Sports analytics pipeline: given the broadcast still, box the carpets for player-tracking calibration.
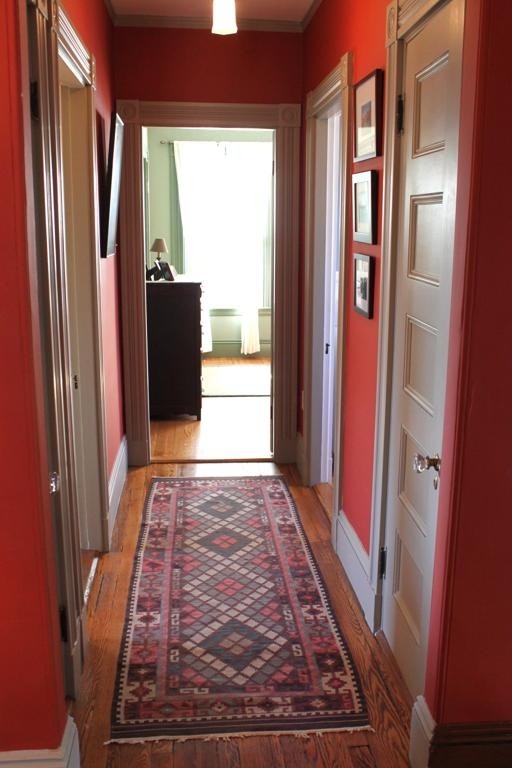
[109,475,373,740]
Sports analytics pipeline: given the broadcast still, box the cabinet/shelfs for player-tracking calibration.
[145,279,202,421]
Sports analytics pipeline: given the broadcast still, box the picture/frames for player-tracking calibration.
[101,109,125,257]
[350,68,384,320]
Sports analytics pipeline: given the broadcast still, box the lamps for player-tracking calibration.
[150,238,168,261]
